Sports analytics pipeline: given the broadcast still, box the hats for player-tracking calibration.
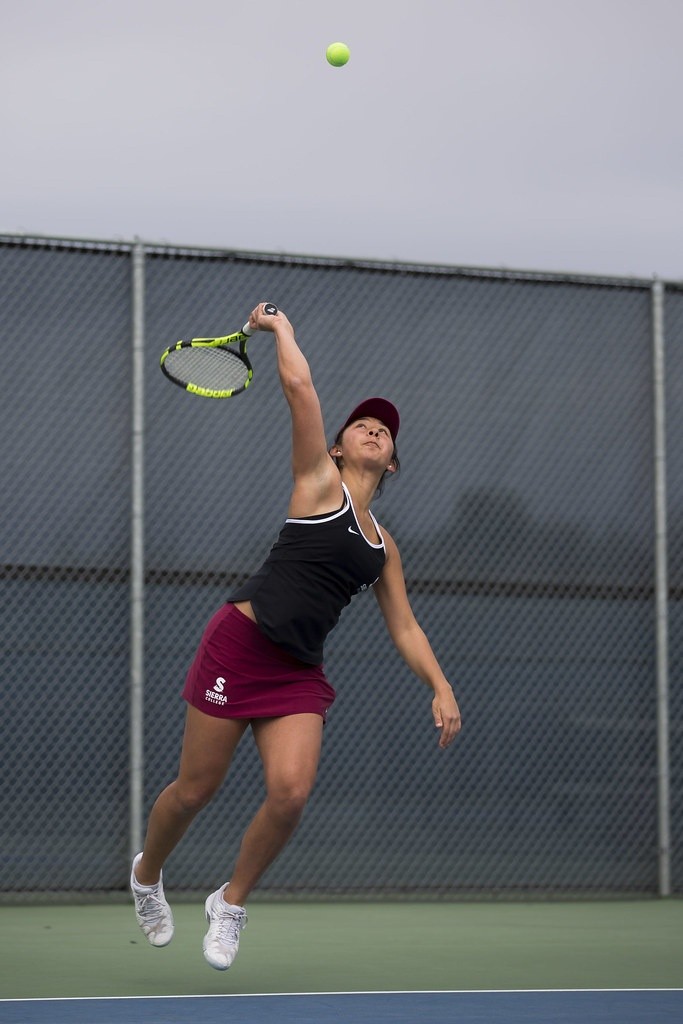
[336,398,400,446]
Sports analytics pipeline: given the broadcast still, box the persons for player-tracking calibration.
[130,302,461,971]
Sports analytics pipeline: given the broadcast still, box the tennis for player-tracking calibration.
[324,40,350,67]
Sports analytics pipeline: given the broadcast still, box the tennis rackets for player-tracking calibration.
[157,303,278,401]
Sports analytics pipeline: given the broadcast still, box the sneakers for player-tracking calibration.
[130,851,174,947]
[202,882,250,971]
